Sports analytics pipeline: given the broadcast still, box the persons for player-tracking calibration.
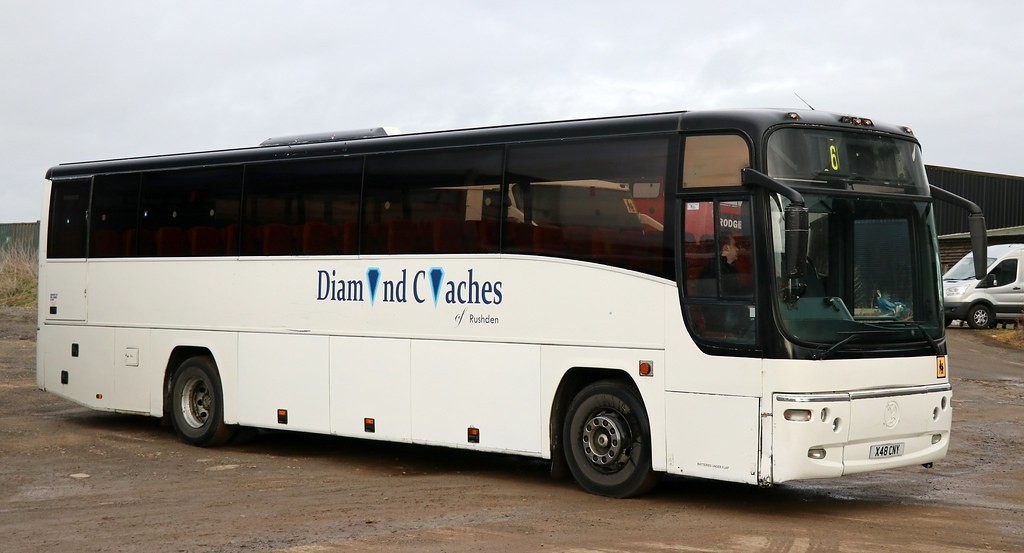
[700,238,755,338]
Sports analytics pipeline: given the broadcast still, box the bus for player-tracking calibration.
[35,108,987,495]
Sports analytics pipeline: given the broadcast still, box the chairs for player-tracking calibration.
[92,217,751,338]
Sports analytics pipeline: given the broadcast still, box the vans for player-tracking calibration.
[941,243,1024,331]
